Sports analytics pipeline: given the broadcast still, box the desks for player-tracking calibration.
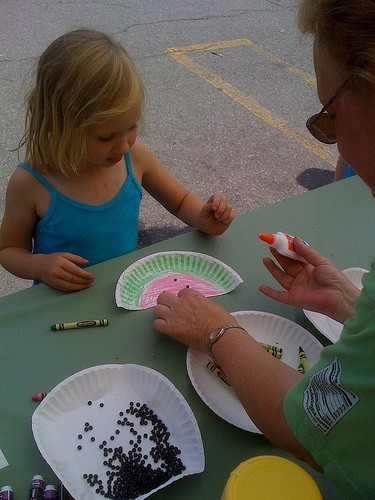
[0,175,375,500]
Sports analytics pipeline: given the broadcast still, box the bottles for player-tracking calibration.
[258,232,311,263]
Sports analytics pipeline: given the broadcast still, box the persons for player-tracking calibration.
[0,30,235,292]
[152,0,375,500]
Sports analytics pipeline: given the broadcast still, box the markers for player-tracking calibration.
[29,474,58,499]
[1,485,14,500]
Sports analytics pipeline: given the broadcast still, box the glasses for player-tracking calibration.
[306,75,356,144]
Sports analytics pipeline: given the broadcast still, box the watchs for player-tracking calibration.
[206,323,247,365]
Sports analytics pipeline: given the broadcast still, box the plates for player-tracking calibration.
[32,365,205,500]
[302,267,370,344]
[115,250,243,310]
[186,311,324,434]
